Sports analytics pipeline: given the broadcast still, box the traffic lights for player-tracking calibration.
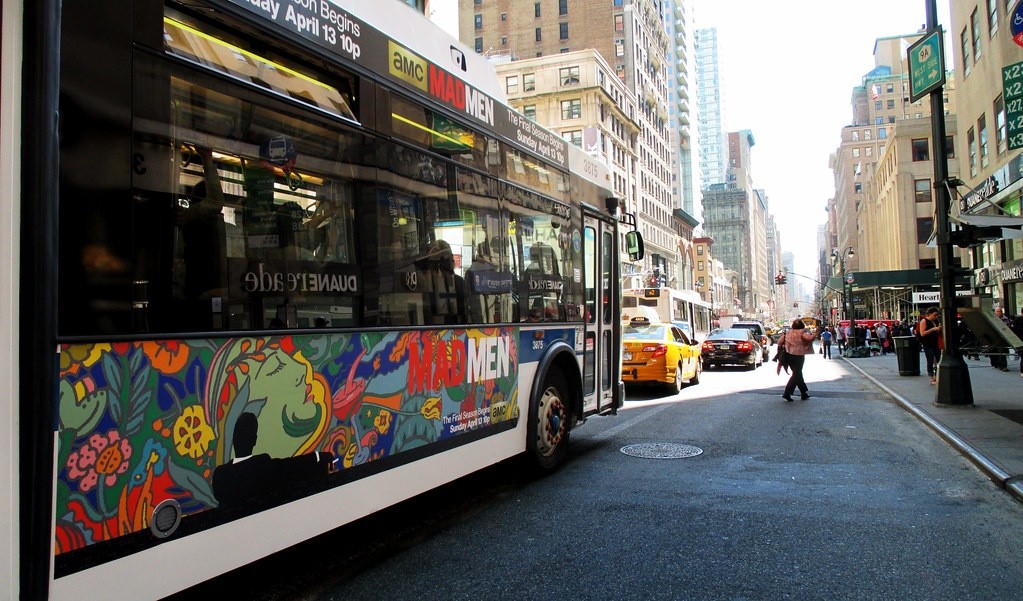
[778,274,783,284]
[794,303,797,307]
[775,275,780,285]
[649,275,657,287]
[782,275,787,284]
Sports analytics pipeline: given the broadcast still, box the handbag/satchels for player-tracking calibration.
[938,333,944,349]
[884,340,889,347]
[819,348,822,354]
[778,348,786,365]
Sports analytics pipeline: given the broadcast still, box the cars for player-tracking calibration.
[763,318,797,343]
[700,327,765,371]
[619,320,703,396]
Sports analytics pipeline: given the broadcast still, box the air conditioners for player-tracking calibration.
[615,39,621,46]
[616,65,622,70]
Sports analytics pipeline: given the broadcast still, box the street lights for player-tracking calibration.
[846,272,856,349]
[829,245,855,320]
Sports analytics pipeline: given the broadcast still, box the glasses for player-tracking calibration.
[934,314,938,316]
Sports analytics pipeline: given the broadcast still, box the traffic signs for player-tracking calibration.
[907,24,946,103]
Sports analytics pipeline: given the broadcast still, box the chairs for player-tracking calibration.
[172,139,567,329]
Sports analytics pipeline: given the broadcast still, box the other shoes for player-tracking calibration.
[823,356,826,359]
[930,379,936,386]
[829,357,831,359]
[801,393,810,400]
[1020,372,1023,377]
[781,393,794,402]
[999,368,1009,372]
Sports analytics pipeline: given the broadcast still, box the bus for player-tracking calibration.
[0,1,648,600]
[800,317,821,339]
[621,286,718,355]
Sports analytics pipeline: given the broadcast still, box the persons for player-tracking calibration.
[864,326,871,346]
[139,133,223,332]
[891,322,900,337]
[984,306,1023,377]
[278,201,334,250]
[826,323,830,331]
[182,178,239,333]
[524,242,581,323]
[465,241,519,321]
[835,323,846,356]
[860,325,866,346]
[58,128,103,337]
[865,305,873,319]
[870,326,878,338]
[816,325,825,340]
[427,238,465,323]
[855,323,860,346]
[885,326,894,353]
[777,318,815,401]
[821,328,832,359]
[84,125,154,335]
[901,319,913,336]
[876,322,888,355]
[841,323,851,341]
[236,165,293,329]
[307,260,363,328]
[920,307,942,384]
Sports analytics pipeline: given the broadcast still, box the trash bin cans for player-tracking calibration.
[847,337,854,350]
[892,336,920,376]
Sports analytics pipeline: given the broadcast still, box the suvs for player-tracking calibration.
[732,320,771,363]
